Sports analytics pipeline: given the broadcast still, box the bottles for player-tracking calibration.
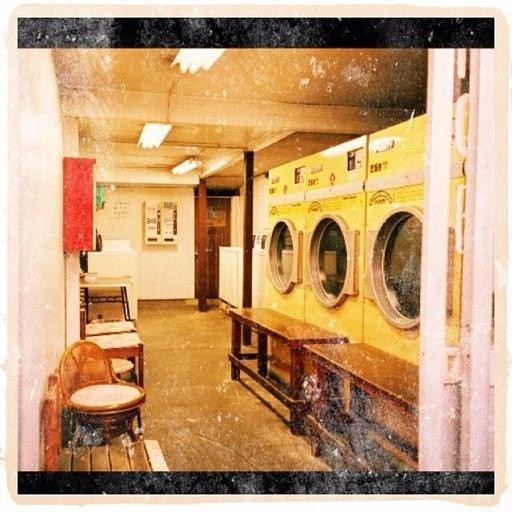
[96,228,103,251]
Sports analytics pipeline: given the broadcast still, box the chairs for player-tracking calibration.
[60,339,147,443]
[83,307,137,333]
[80,310,144,388]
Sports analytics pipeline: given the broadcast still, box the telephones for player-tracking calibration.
[96,228,102,252]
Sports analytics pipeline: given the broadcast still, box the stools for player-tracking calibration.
[111,357,138,384]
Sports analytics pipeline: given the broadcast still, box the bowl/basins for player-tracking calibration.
[83,273,97,283]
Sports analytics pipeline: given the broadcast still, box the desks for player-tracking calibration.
[229,306,349,436]
[301,342,418,471]
[78,276,132,320]
[40,374,167,472]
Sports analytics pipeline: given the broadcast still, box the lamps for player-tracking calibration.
[137,124,172,152]
[167,49,230,75]
[170,158,204,174]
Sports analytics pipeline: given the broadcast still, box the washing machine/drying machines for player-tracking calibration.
[219,236,264,310]
[265,156,306,323]
[305,132,370,345]
[364,101,468,366]
